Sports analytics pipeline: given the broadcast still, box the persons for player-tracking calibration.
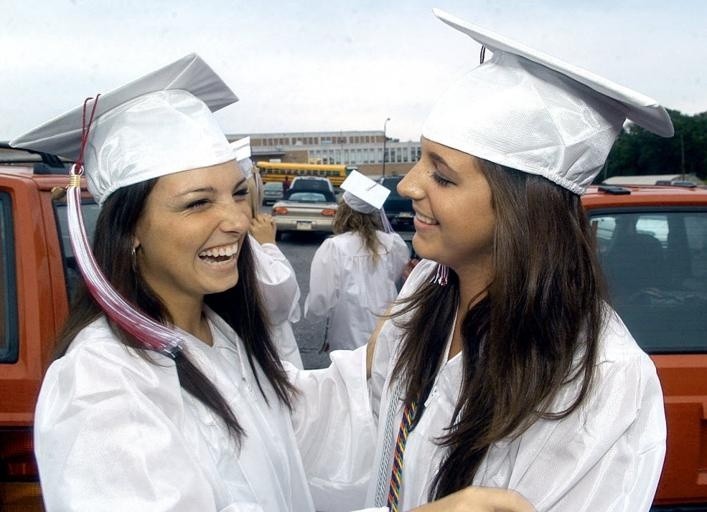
[33,89,536,511]
[235,157,306,372]
[359,50,669,511]
[303,190,408,358]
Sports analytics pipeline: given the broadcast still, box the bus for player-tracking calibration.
[256,161,346,195]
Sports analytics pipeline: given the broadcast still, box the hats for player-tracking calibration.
[8,52,260,207]
[340,169,392,214]
[421,6,675,194]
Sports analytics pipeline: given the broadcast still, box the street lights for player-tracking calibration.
[382,118,391,176]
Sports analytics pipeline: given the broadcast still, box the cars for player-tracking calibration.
[0,140,101,511]
[271,188,339,242]
[289,175,335,198]
[577,179,705,506]
[376,176,414,232]
[262,180,284,205]
